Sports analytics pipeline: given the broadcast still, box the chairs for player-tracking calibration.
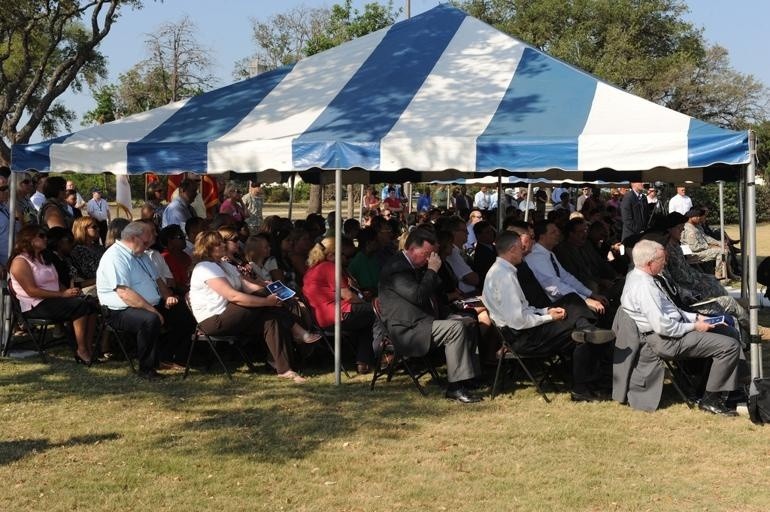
[297,291,353,379]
[487,309,564,402]
[3,278,55,364]
[82,285,138,375]
[370,297,441,399]
[183,292,259,385]
[618,307,698,411]
[687,260,717,275]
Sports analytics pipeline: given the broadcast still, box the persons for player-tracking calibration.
[3,172,767,417]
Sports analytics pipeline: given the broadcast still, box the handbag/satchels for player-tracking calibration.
[749,379,770,423]
[673,358,709,400]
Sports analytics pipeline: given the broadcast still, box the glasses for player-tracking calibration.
[171,234,186,239]
[23,179,29,184]
[92,225,97,229]
[227,236,239,243]
[39,233,50,238]
[0,186,9,191]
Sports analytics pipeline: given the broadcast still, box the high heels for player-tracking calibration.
[74,351,97,365]
[285,372,300,381]
[294,334,322,344]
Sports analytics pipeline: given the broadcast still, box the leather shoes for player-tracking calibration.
[358,364,375,374]
[572,327,616,344]
[699,401,739,417]
[138,370,169,382]
[571,391,606,402]
[446,388,480,403]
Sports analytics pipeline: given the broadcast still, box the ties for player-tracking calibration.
[550,254,560,277]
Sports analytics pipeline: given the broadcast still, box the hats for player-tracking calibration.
[92,188,102,192]
[686,206,706,219]
[665,211,689,227]
